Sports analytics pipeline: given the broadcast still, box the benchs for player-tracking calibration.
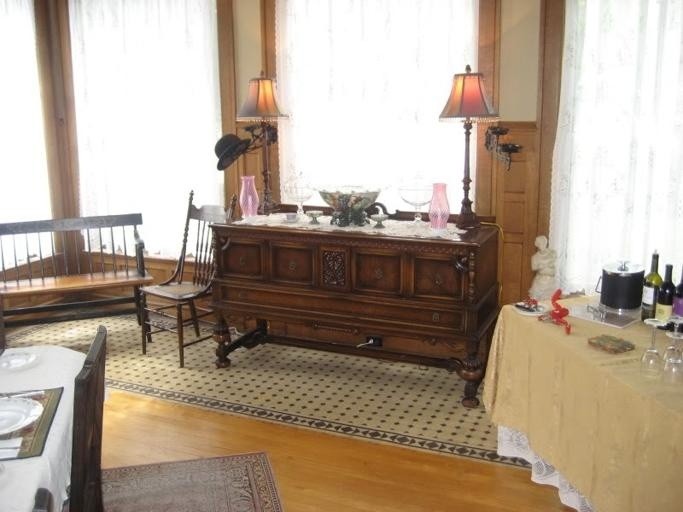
[0,214,155,346]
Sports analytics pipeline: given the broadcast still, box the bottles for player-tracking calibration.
[673,264,683,333]
[655,263,675,331]
[641,252,662,320]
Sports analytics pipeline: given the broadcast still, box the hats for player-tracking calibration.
[214,133,251,171]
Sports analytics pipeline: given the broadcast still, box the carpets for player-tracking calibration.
[98,449,284,511]
[4,311,531,469]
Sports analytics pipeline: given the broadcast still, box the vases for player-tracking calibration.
[237,174,259,219]
[427,183,450,231]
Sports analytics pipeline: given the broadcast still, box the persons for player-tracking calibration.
[527,234,560,301]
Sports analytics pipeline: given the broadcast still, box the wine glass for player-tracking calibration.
[369,214,388,229]
[639,317,682,372]
[398,188,434,226]
[282,185,316,224]
[305,210,323,224]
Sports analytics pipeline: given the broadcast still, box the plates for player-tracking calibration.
[0,398,44,436]
[514,300,551,317]
[0,351,37,369]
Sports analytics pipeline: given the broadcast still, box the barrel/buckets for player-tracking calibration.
[596,263,646,308]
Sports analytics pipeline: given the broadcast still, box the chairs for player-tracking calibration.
[69,325,108,512]
[139,189,236,369]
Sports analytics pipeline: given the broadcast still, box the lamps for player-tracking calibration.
[234,69,290,215]
[436,64,503,230]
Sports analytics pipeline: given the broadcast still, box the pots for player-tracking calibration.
[592,257,641,313]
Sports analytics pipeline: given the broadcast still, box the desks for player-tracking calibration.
[481,292,683,510]
[207,211,503,408]
